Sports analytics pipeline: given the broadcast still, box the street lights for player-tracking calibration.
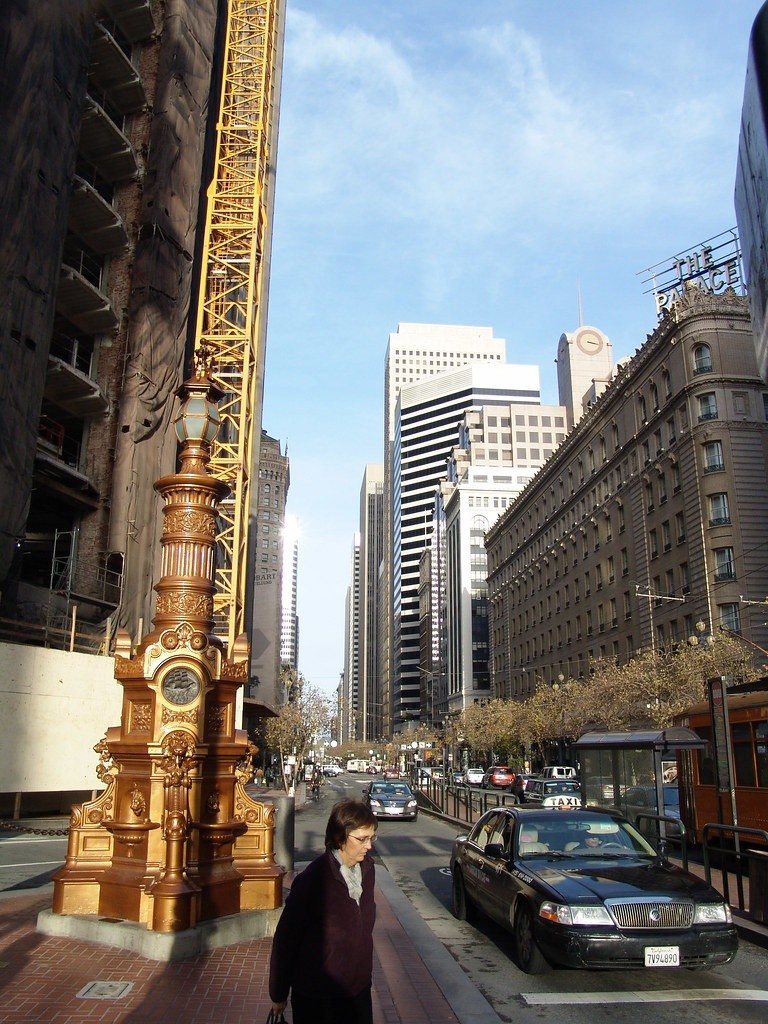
[553,674,571,766]
[688,621,716,701]
[442,715,452,772]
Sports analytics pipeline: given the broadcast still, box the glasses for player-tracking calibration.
[348,835,378,843]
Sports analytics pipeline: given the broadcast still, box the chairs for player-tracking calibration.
[518,825,549,855]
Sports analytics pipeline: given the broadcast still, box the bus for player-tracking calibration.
[369,761,388,772]
[346,760,369,773]
[670,691,768,847]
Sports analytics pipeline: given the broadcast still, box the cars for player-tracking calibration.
[383,769,400,780]
[362,781,418,822]
[539,766,575,779]
[462,769,485,788]
[322,765,344,775]
[323,769,337,777]
[447,773,465,785]
[607,783,681,837]
[578,776,631,800]
[510,774,538,794]
[481,766,515,790]
[514,778,582,804]
[367,767,377,774]
[449,806,739,975]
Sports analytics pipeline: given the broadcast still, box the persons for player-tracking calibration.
[255,765,323,793]
[545,784,572,793]
[572,834,630,850]
[269,800,379,1024]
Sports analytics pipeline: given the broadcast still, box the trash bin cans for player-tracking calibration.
[305,782,314,800]
[272,796,296,871]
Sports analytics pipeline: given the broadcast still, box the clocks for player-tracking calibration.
[577,330,603,354]
